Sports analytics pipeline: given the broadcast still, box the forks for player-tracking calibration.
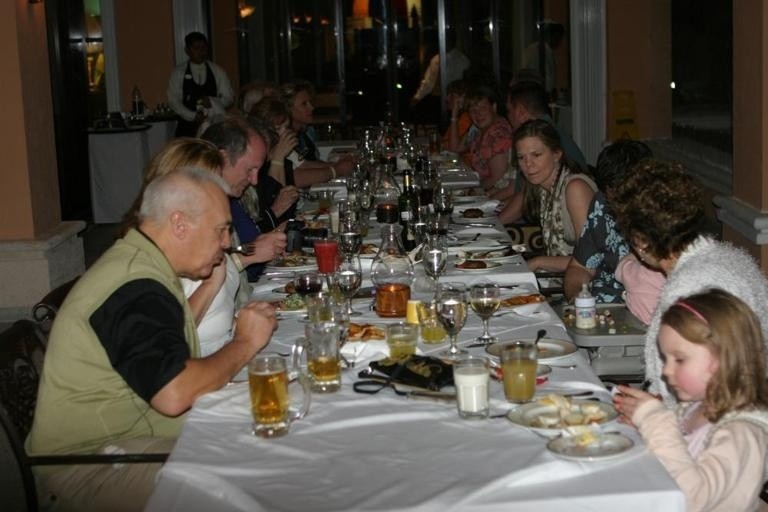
[227,242,256,256]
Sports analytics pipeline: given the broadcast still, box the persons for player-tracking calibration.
[446,85,514,197]
[193,114,287,281]
[164,30,232,138]
[610,290,768,507]
[244,103,299,217]
[603,156,768,400]
[407,30,471,133]
[439,79,477,151]
[489,80,590,227]
[239,122,300,233]
[507,119,595,272]
[614,252,664,326]
[278,79,319,161]
[117,141,239,359]
[256,105,357,189]
[24,165,280,506]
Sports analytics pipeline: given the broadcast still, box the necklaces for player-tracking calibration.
[541,179,551,235]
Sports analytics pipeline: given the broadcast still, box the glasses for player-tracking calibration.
[467,104,490,116]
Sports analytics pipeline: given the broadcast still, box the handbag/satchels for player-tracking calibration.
[351,352,454,397]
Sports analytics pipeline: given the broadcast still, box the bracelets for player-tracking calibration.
[327,164,337,179]
[450,116,458,122]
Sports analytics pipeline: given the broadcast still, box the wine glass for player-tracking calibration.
[341,233,362,272]
[338,271,361,317]
[436,282,469,358]
[294,271,323,321]
[423,234,449,301]
[343,211,356,232]
[433,187,454,216]
[352,178,360,201]
[469,283,500,345]
[430,217,449,235]
[453,355,490,420]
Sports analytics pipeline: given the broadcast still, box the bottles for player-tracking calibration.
[373,163,403,225]
[370,224,416,316]
[397,168,422,252]
[131,85,146,123]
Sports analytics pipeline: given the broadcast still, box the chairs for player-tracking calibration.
[32,274,138,339]
[0,319,170,512]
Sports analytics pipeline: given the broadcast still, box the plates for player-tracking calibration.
[451,200,501,223]
[507,398,619,440]
[233,303,307,324]
[452,230,508,242]
[546,423,648,462]
[303,209,329,220]
[486,338,579,360]
[453,261,504,274]
[266,255,320,271]
[459,254,521,261]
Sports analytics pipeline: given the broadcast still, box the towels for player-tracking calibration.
[485,352,608,404]
[204,94,226,115]
[341,342,389,365]
[437,271,540,314]
[188,378,308,422]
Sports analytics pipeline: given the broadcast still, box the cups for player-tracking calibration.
[384,323,418,365]
[415,303,447,344]
[313,241,337,272]
[499,343,537,403]
[247,351,311,438]
[291,321,342,394]
